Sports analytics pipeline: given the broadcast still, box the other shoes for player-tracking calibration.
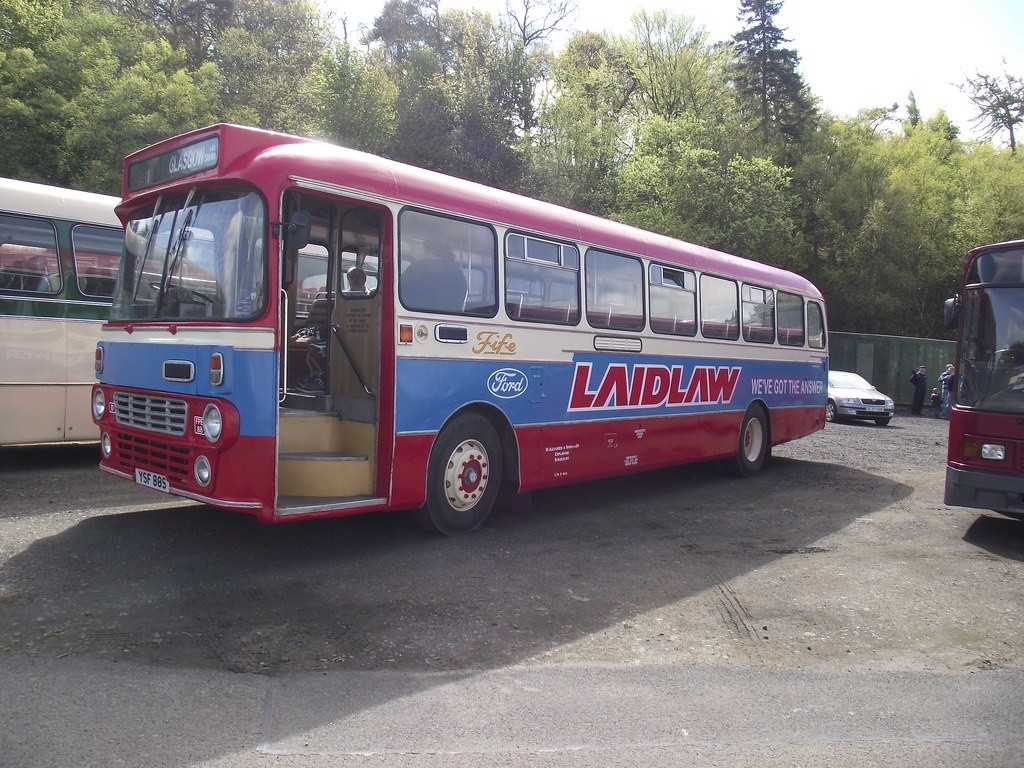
[912,412,924,415]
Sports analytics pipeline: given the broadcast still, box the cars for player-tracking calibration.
[826,370,895,427]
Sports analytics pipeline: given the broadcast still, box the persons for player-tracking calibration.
[910,366,926,415]
[401,240,467,311]
[346,266,371,295]
[931,364,955,420]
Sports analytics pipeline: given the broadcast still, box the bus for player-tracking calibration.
[940,236,1023,529]
[0,174,412,450]
[89,123,832,539]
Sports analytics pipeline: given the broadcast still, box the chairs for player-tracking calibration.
[465,298,806,347]
[289,291,330,374]
[4,255,120,298]
